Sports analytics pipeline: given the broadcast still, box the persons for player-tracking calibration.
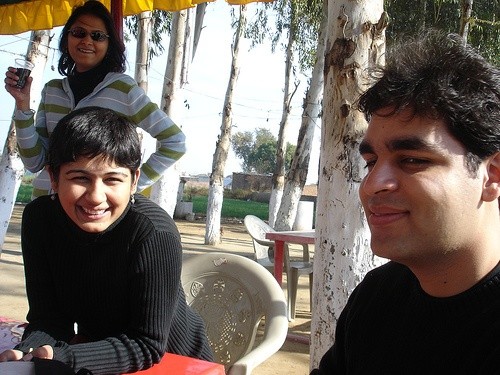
[308,29,500,375]
[3,1,185,194]
[0,107,214,375]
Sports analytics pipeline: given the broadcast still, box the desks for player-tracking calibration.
[0,315,228,375]
[265,230,316,287]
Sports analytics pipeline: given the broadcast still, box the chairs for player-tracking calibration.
[179,251,290,375]
[243,214,313,321]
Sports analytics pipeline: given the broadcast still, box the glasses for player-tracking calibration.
[67,27,110,42]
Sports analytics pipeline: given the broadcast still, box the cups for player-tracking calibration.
[10,59,35,90]
